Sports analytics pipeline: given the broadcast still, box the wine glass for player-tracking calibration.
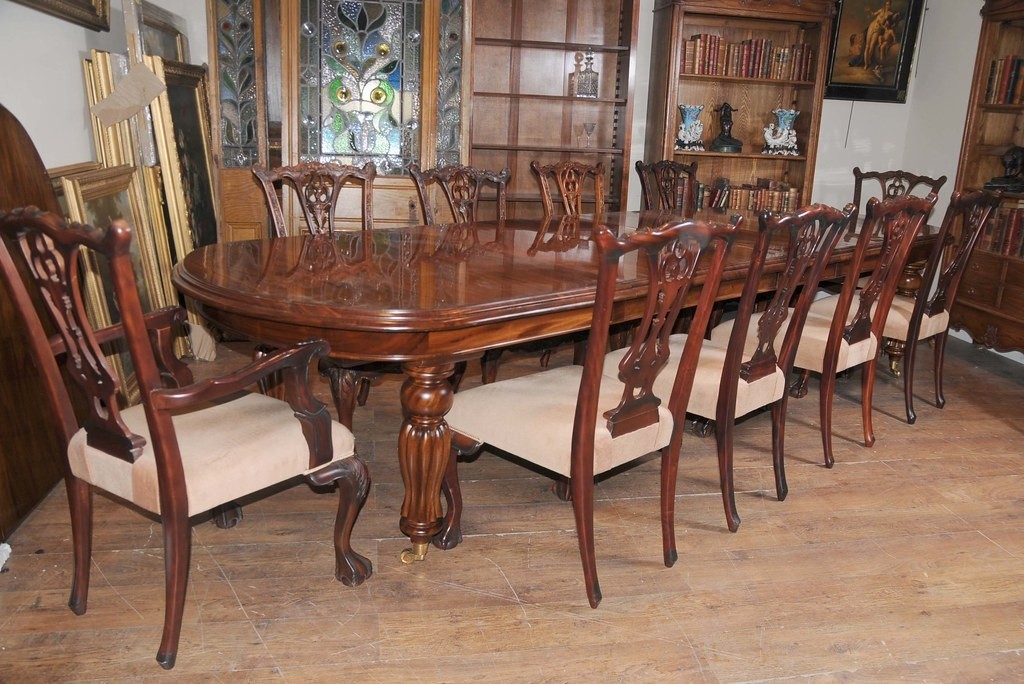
[583,123,597,148]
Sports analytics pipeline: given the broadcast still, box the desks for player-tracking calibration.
[172,220,939,563]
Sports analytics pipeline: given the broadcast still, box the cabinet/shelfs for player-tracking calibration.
[458,0,640,217]
[640,0,836,212]
[928,0,1023,354]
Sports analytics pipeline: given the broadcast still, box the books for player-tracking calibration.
[676,176,799,213]
[984,54,1024,104]
[974,198,1024,259]
[680,33,812,81]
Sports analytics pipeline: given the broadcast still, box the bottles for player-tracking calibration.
[576,49,599,99]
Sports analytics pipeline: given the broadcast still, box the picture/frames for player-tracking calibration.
[825,1,923,103]
[12,0,224,411]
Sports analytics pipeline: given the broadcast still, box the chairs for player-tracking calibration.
[251,162,996,609]
[1,202,374,669]
